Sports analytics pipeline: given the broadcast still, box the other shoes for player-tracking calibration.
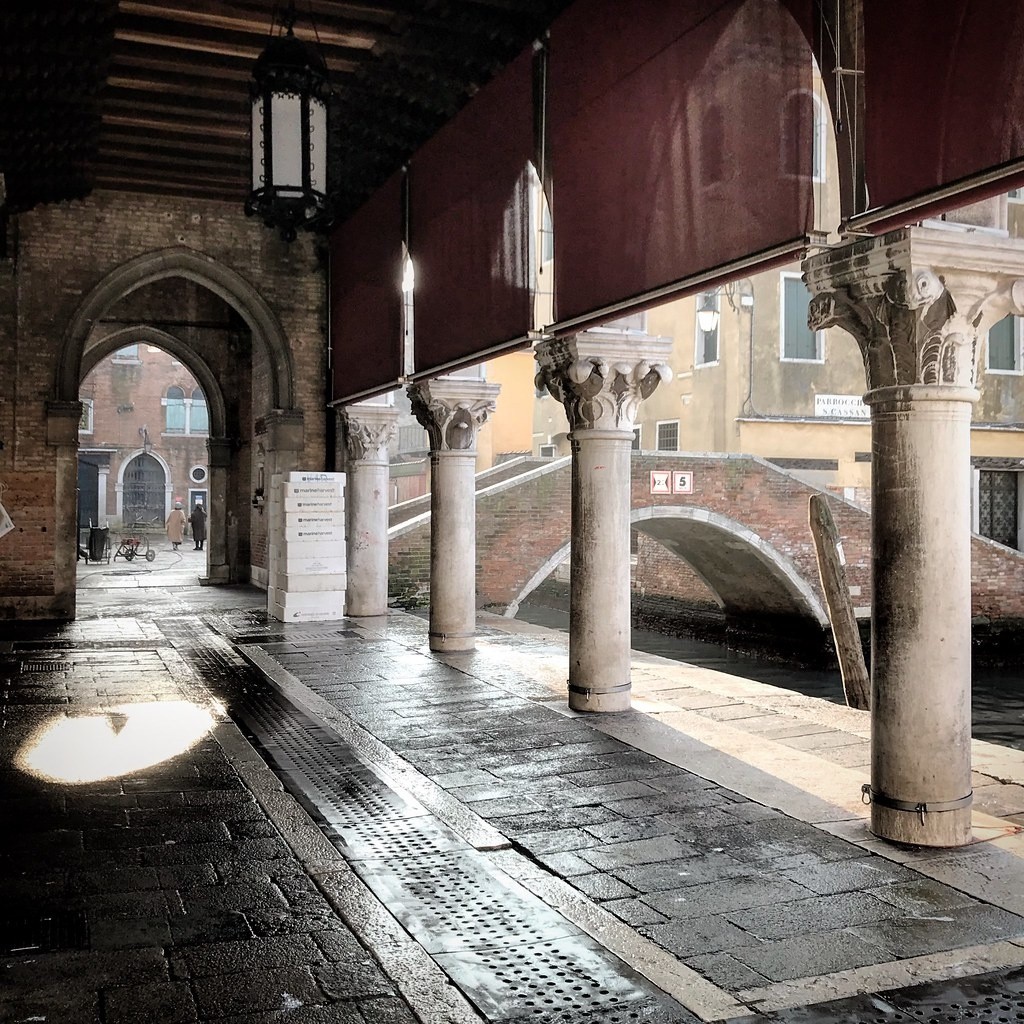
[173,547,178,551]
[199,547,203,550]
[193,547,199,550]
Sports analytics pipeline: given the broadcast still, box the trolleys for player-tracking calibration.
[114,516,159,561]
[85,518,112,565]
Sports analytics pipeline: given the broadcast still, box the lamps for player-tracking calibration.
[244,0,338,244]
[139,428,153,452]
[696,292,720,339]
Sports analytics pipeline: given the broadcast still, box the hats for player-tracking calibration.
[175,502,182,508]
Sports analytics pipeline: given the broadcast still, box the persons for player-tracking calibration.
[188,504,207,551]
[166,502,186,551]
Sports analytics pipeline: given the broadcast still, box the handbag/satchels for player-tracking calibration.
[188,517,192,522]
